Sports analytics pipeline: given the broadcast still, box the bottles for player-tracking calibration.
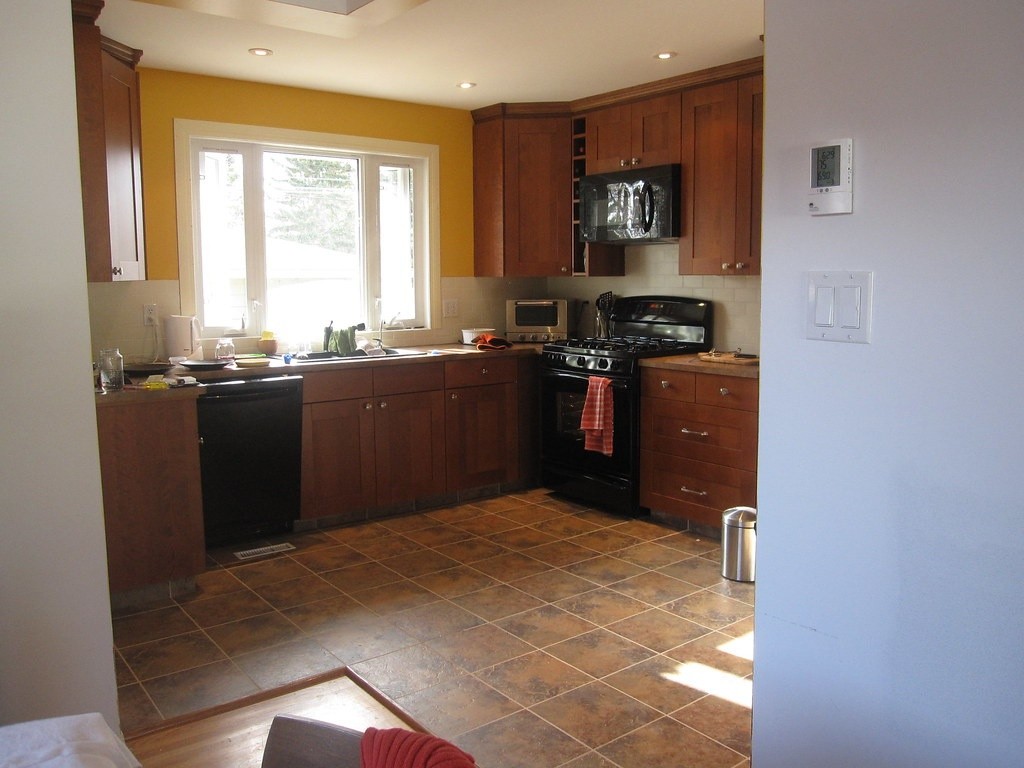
[214,337,235,360]
[99,347,125,393]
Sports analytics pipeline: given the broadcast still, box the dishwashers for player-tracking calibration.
[194,376,303,543]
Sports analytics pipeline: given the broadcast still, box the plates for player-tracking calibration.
[234,358,270,367]
[178,359,229,369]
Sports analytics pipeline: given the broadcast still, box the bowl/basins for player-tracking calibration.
[124,364,176,383]
[461,327,496,346]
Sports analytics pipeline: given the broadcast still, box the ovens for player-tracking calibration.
[539,368,639,504]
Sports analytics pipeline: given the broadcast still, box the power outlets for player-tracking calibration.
[442,298,459,318]
[143,304,159,326]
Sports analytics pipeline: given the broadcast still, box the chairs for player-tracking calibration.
[260,713,474,768]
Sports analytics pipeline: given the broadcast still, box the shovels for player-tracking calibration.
[600,291,612,310]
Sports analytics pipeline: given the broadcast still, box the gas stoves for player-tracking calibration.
[542,295,714,376]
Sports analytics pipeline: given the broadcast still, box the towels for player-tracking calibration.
[579,375,614,456]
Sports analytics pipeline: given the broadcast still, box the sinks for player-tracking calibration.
[292,351,341,361]
[336,348,419,357]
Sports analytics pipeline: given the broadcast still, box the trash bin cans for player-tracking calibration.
[721,506,756,582]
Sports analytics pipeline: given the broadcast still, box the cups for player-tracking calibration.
[258,340,278,355]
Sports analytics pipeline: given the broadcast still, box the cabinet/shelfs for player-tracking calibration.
[292,362,446,534]
[444,356,519,508]
[470,99,573,279]
[72,0,150,284]
[678,54,764,276]
[571,97,626,278]
[640,366,759,542]
[586,75,682,176]
[95,395,205,594]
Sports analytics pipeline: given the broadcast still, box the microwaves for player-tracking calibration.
[577,163,680,245]
[504,298,576,343]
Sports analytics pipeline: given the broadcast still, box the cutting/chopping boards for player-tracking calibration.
[698,351,759,366]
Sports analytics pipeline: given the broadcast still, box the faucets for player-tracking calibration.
[322,322,365,351]
